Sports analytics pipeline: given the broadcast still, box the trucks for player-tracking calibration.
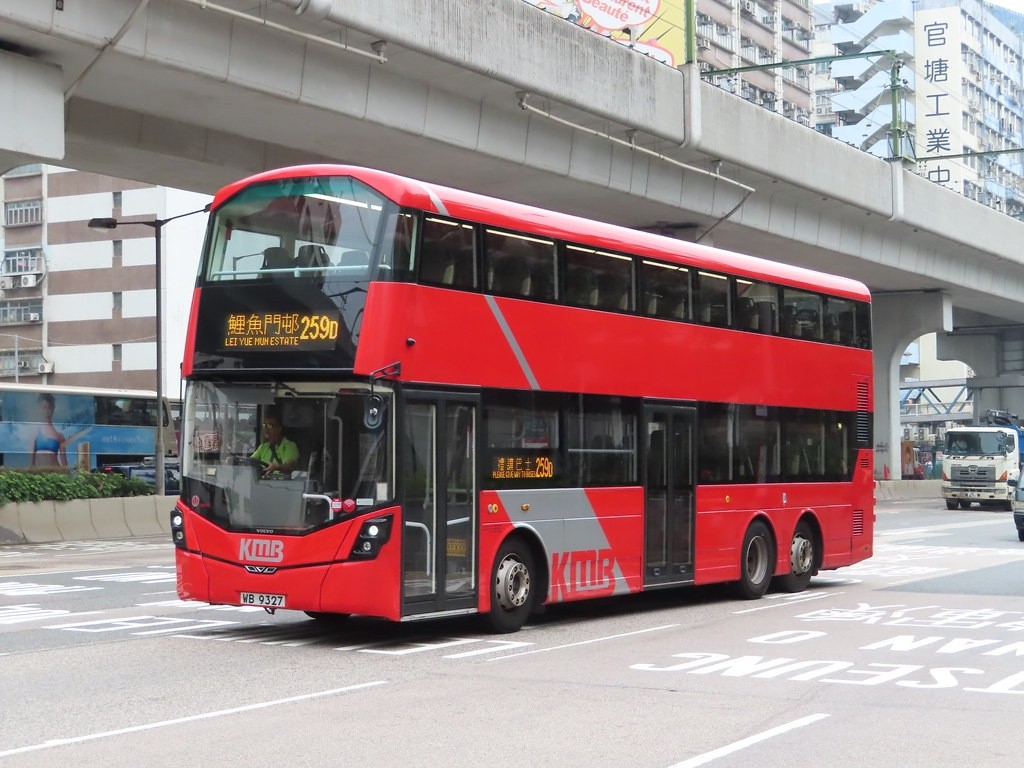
[901,441,926,480]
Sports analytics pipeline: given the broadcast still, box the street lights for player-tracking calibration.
[86,202,212,496]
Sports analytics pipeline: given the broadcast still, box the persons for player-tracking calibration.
[226,414,300,479]
[30,393,70,474]
[952,435,967,450]
[925,459,933,478]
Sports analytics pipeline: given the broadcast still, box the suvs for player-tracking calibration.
[95,461,181,497]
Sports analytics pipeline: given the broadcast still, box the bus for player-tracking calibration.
[169,163,876,636]
[162,397,260,460]
[0,382,179,482]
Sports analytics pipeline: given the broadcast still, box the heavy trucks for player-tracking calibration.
[940,424,1024,511]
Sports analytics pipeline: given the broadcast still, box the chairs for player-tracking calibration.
[579,428,841,503]
[256,243,859,346]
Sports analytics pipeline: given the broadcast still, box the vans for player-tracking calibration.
[1012,466,1024,542]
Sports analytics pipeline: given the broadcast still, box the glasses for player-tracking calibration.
[262,423,277,429]
